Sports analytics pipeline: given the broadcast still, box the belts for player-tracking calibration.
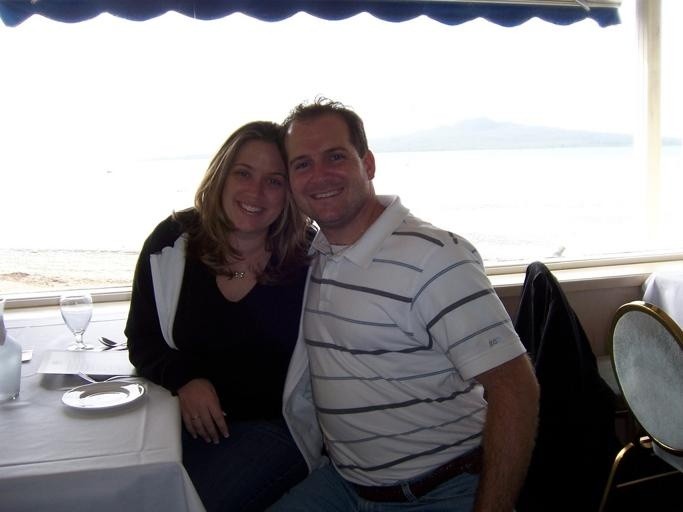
[350,446,481,503]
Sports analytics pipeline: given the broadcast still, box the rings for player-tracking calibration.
[191,413,200,420]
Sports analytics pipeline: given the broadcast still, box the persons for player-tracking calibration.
[271,101,542,511]
[124,123,319,511]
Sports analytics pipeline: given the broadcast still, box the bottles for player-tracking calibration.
[0,299,23,402]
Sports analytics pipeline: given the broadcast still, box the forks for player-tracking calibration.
[77,370,145,384]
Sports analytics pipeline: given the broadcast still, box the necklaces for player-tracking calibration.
[232,268,249,282]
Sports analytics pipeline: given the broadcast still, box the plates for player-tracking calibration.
[60,380,146,414]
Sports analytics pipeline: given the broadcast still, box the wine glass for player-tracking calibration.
[59,289,94,351]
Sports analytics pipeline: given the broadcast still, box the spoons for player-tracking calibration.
[98,336,127,348]
